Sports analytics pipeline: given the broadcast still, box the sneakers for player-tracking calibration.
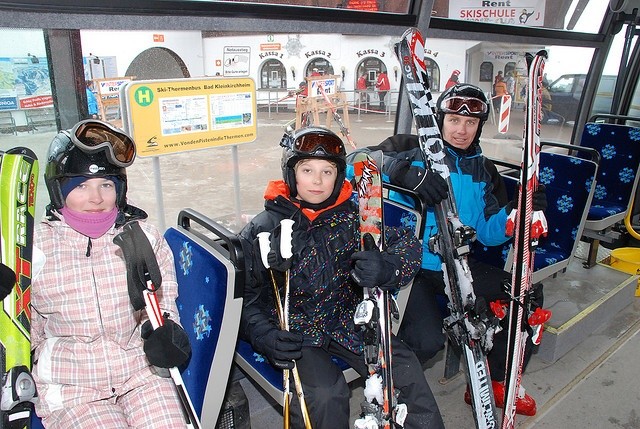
[464,379,536,416]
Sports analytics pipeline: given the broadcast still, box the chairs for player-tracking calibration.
[570,114,640,231]
[30,208,246,429]
[236,181,427,409]
[432,156,522,384]
[528,142,602,286]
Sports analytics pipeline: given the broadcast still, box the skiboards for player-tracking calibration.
[396,27,548,429]
[354,150,397,429]
[349,105,390,113]
[317,85,358,149]
[1,147,39,429]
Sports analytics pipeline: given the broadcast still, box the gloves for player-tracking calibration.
[349,232,401,291]
[141,311,191,368]
[389,155,449,206]
[510,183,548,212]
[245,313,303,369]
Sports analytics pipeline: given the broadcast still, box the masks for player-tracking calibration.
[59,203,118,239]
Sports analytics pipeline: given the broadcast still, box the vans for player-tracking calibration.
[523,73,618,124]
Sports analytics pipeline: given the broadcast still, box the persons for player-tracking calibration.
[446,71,460,91]
[495,71,504,84]
[374,71,389,111]
[505,73,515,95]
[494,79,507,113]
[31,120,192,429]
[357,73,372,111]
[238,127,445,429]
[346,85,553,416]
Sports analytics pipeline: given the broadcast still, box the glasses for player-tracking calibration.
[293,130,343,155]
[71,118,136,167]
[440,95,488,115]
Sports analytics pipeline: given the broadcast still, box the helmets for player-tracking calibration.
[279,126,347,211]
[44,127,128,224]
[435,82,490,147]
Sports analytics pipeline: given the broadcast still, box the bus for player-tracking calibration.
[0,0,640,429]
